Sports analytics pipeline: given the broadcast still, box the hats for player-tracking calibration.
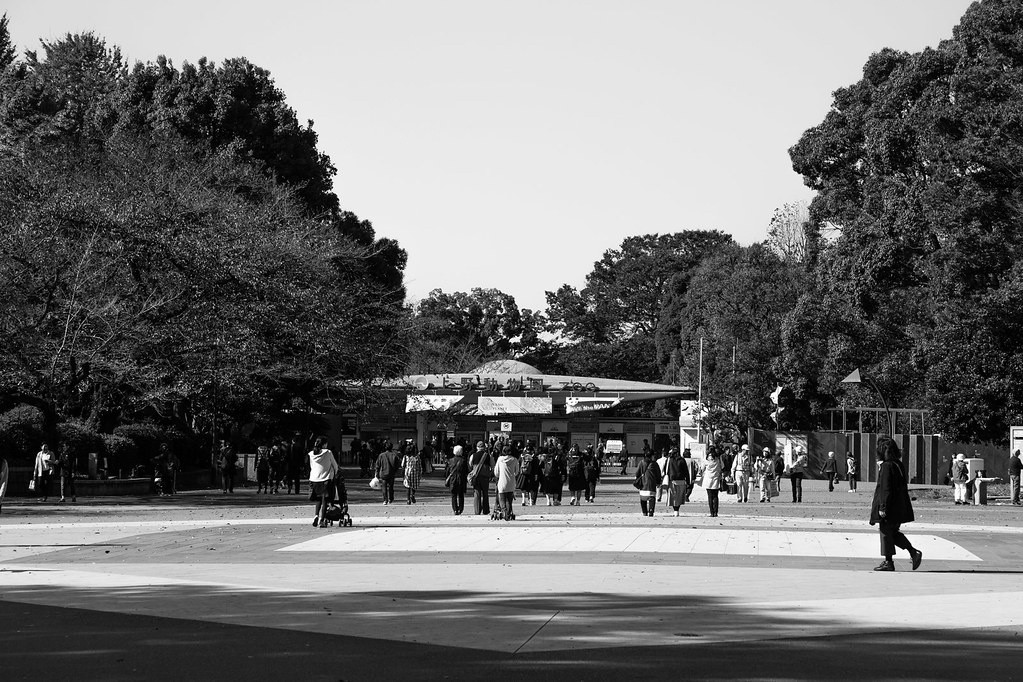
[762,447,770,453]
[742,444,750,450]
[794,445,803,453]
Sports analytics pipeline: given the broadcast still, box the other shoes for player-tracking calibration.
[736,486,856,504]
[1013,500,1021,505]
[955,501,962,505]
[37,497,76,505]
[223,487,594,528]
[963,500,970,505]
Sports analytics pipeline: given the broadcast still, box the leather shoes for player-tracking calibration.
[874,561,895,572]
[910,550,922,570]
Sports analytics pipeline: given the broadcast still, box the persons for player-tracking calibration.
[218,439,239,493]
[946,452,971,505]
[154,443,181,497]
[869,436,921,572]
[255,430,857,527]
[33,441,55,502]
[1007,450,1023,508]
[46,442,76,502]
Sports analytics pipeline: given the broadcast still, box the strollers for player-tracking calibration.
[323,468,352,527]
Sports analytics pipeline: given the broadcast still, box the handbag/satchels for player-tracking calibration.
[29,480,35,491]
[834,475,839,483]
[964,474,968,480]
[445,474,451,487]
[633,476,643,490]
[662,475,669,489]
[766,472,773,480]
[369,476,381,490]
[467,470,477,486]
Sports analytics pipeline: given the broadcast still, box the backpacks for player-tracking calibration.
[583,458,596,482]
[521,453,534,476]
[543,454,558,476]
[567,455,582,475]
